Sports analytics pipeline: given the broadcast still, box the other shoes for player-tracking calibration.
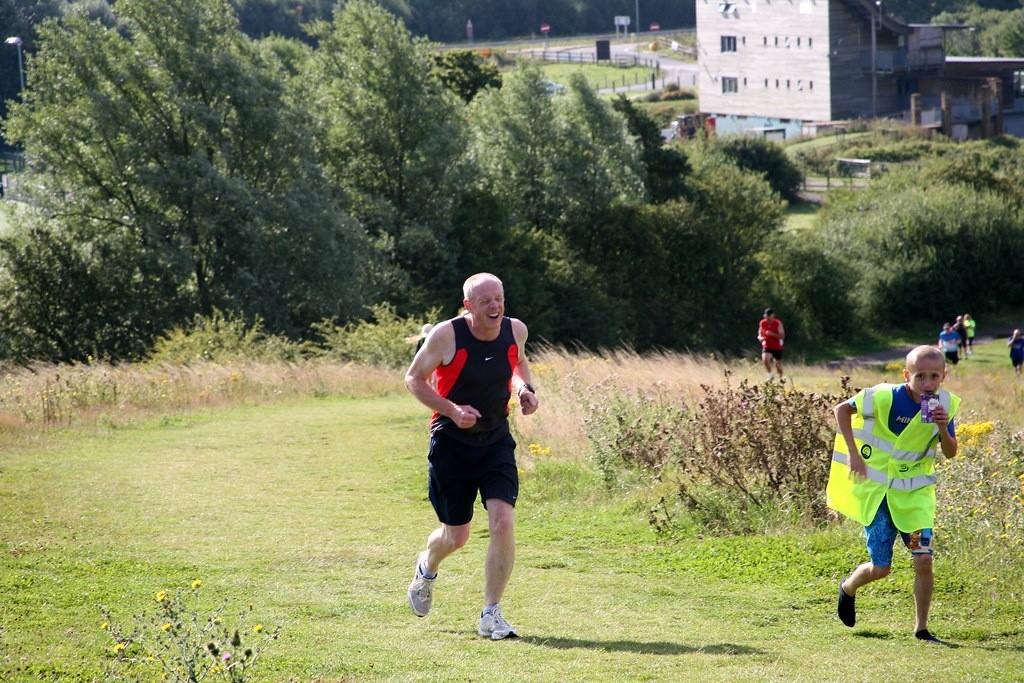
[838,578,855,627]
[914,629,946,643]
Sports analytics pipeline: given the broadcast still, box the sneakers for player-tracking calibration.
[478,604,518,640]
[408,551,438,617]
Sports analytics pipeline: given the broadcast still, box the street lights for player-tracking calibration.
[4,36,25,104]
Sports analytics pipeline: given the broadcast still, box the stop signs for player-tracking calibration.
[650,22,660,33]
[540,24,550,33]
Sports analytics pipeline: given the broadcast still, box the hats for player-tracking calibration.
[763,307,774,317]
[421,324,434,339]
[943,322,950,329]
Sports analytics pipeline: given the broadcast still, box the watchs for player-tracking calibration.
[518,383,535,395]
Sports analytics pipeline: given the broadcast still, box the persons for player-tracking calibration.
[952,316,967,355]
[759,308,785,378]
[1007,329,1024,374]
[415,324,436,391]
[825,345,962,641]
[938,323,961,370]
[404,274,539,639]
[963,314,975,353]
